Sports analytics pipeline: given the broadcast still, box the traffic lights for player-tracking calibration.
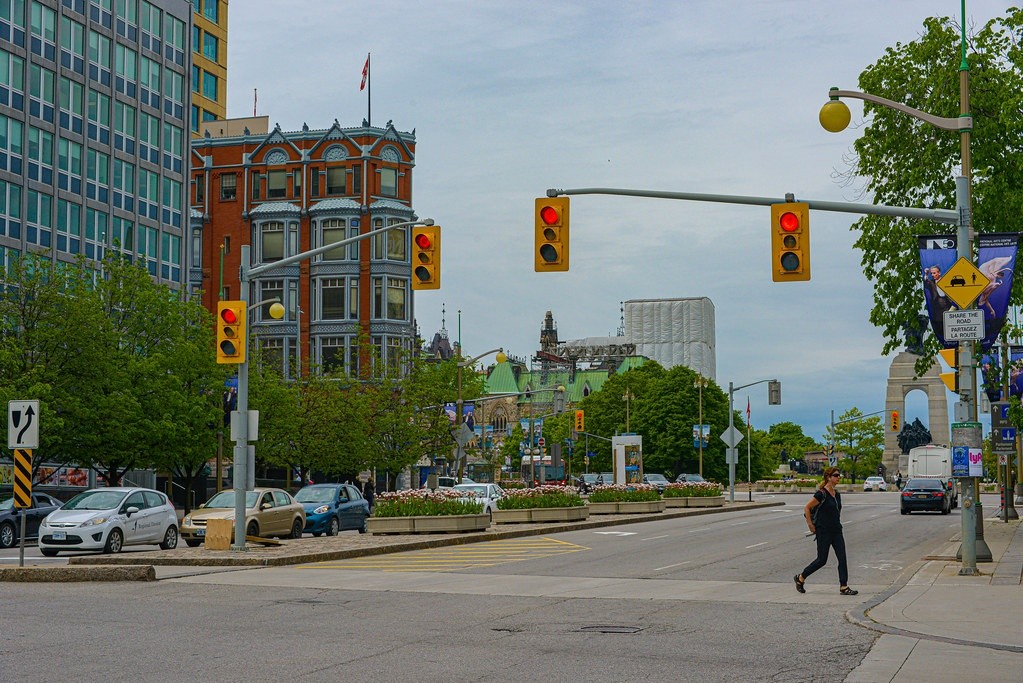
[574,410,585,432]
[938,347,959,394]
[412,225,442,291]
[770,203,811,282]
[822,445,834,456]
[533,197,570,271]
[891,411,901,431]
[216,301,245,365]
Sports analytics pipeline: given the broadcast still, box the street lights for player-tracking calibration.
[819,86,981,580]
[621,385,636,432]
[456,343,506,481]
[693,368,708,476]
[233,297,285,549]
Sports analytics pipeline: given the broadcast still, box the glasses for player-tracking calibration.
[830,473,841,477]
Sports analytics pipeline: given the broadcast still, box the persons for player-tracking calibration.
[437,468,469,480]
[781,448,789,464]
[1011,471,1018,493]
[979,255,1013,319]
[895,469,902,493]
[295,470,373,510]
[576,472,603,494]
[982,363,998,398]
[924,264,957,337]
[223,387,237,416]
[448,411,472,423]
[793,466,858,596]
[1011,358,1023,389]
[261,497,273,507]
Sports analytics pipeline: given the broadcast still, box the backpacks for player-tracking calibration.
[803,490,825,531]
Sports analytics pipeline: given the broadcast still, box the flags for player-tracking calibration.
[360,53,370,91]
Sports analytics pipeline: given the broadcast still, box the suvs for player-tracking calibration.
[676,474,707,483]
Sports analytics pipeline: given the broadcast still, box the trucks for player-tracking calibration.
[906,445,953,481]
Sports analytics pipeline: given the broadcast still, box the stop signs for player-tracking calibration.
[538,438,545,446]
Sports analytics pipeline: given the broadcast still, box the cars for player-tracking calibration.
[38,487,180,555]
[180,488,308,548]
[0,492,66,549]
[566,472,614,492]
[292,484,370,538]
[899,478,951,515]
[644,473,671,495]
[863,477,889,491]
[423,474,506,521]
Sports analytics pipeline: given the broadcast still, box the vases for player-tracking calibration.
[367,497,725,536]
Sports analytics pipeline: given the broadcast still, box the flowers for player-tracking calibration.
[373,490,485,518]
[663,480,724,498]
[587,483,662,503]
[496,484,586,510]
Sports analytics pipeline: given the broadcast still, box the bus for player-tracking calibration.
[522,455,566,487]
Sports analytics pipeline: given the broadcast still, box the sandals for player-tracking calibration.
[839,587,859,595]
[793,574,806,593]
